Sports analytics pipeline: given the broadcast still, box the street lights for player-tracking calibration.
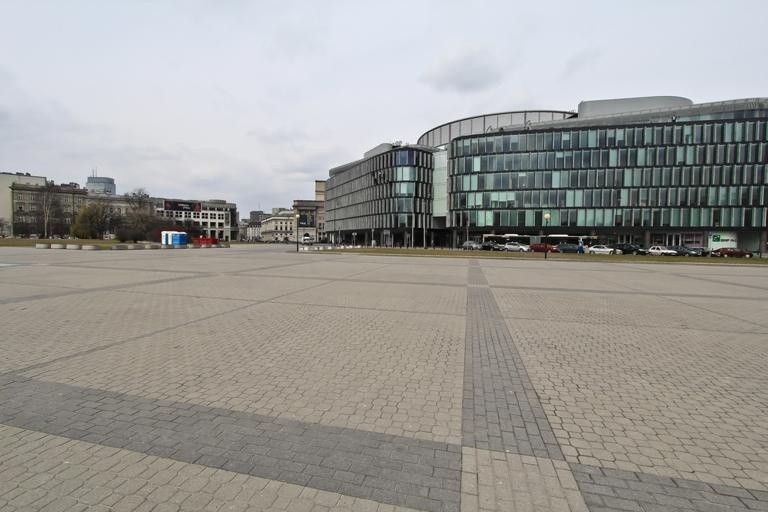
[542,213,550,258]
[295,213,300,253]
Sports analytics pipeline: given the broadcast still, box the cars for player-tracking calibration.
[460,240,755,259]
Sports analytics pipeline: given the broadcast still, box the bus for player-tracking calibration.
[483,233,600,245]
